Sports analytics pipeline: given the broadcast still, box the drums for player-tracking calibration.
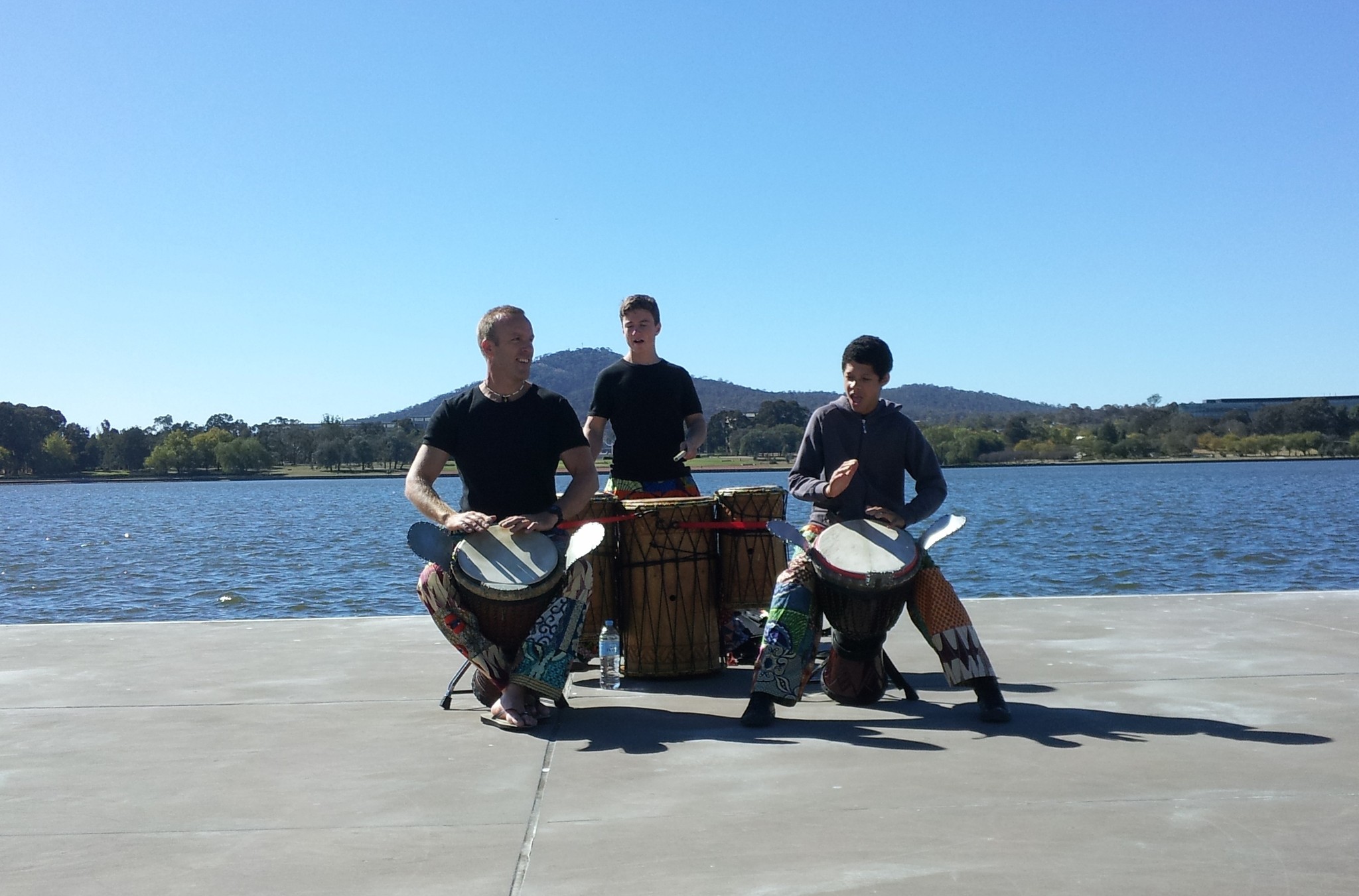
[716,485,789,608]
[450,524,564,710]
[618,496,720,680]
[808,518,923,707]
[555,491,618,661]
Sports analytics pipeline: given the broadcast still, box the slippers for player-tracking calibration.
[524,699,551,718]
[480,708,535,730]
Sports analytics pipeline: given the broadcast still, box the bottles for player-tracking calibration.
[599,620,620,689]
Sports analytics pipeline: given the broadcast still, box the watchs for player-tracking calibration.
[548,505,563,524]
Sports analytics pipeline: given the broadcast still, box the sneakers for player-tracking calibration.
[974,683,1011,722]
[741,692,776,727]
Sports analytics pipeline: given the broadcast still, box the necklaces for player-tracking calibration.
[483,379,525,402]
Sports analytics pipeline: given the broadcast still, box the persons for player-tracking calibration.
[582,294,706,501]
[741,334,1010,729]
[405,305,600,732]
